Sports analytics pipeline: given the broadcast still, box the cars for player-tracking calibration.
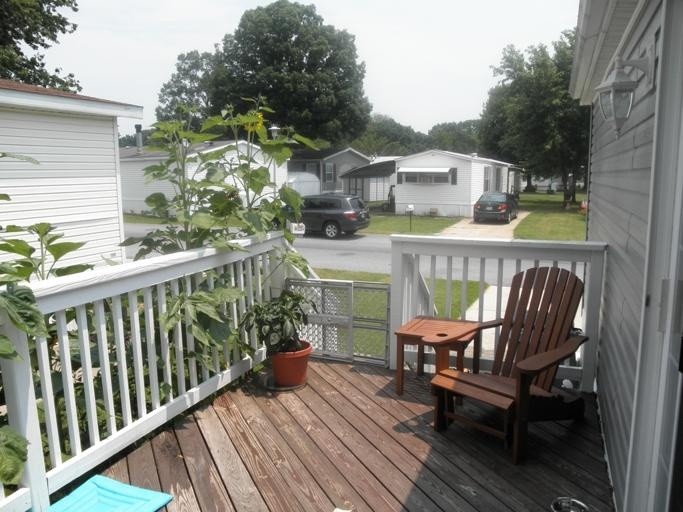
[473,192,519,223]
[269,192,370,239]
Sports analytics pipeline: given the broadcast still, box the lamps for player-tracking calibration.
[594,43,656,141]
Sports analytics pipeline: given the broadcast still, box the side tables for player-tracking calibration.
[394,316,480,406]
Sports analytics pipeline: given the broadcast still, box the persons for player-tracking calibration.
[388,185,395,211]
[564,188,573,208]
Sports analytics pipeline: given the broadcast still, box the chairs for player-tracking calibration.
[421,266,588,464]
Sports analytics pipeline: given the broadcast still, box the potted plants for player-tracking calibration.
[238,289,318,386]
